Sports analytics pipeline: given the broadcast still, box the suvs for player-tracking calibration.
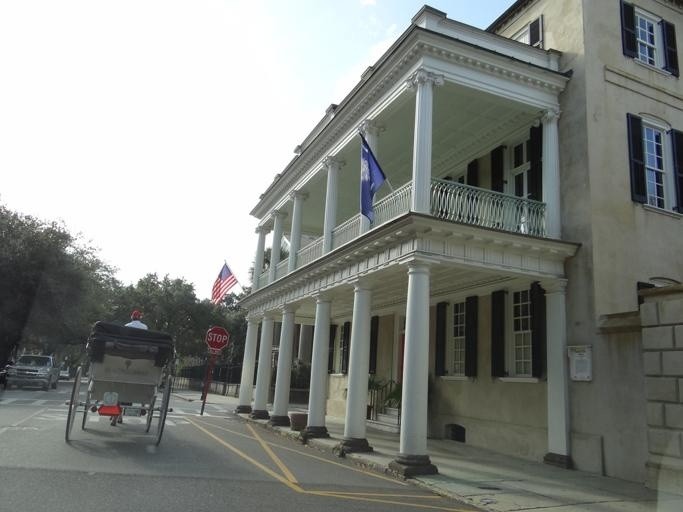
[5,355,60,391]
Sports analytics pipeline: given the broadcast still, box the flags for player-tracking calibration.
[360,136,387,224]
[212,263,238,305]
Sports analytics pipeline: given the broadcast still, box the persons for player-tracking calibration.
[124,311,148,330]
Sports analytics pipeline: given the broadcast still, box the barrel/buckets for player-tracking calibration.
[289,412,308,431]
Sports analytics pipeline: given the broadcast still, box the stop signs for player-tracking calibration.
[203,326,230,350]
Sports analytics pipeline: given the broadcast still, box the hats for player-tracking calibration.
[131,311,141,319]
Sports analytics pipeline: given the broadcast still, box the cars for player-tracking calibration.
[58,364,71,380]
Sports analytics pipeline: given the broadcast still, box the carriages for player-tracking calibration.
[62,320,176,447]
